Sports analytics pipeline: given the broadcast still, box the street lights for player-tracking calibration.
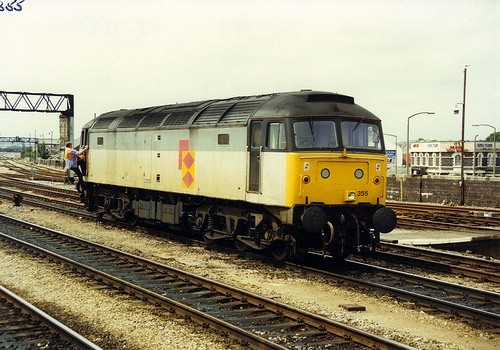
[452,66,468,205]
[471,123,496,180]
[406,110,435,175]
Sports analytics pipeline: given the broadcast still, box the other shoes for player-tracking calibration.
[82,173,86,176]
[79,181,85,184]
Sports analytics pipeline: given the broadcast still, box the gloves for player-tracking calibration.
[84,145,89,152]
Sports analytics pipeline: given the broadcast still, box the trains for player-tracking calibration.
[75,88,397,266]
